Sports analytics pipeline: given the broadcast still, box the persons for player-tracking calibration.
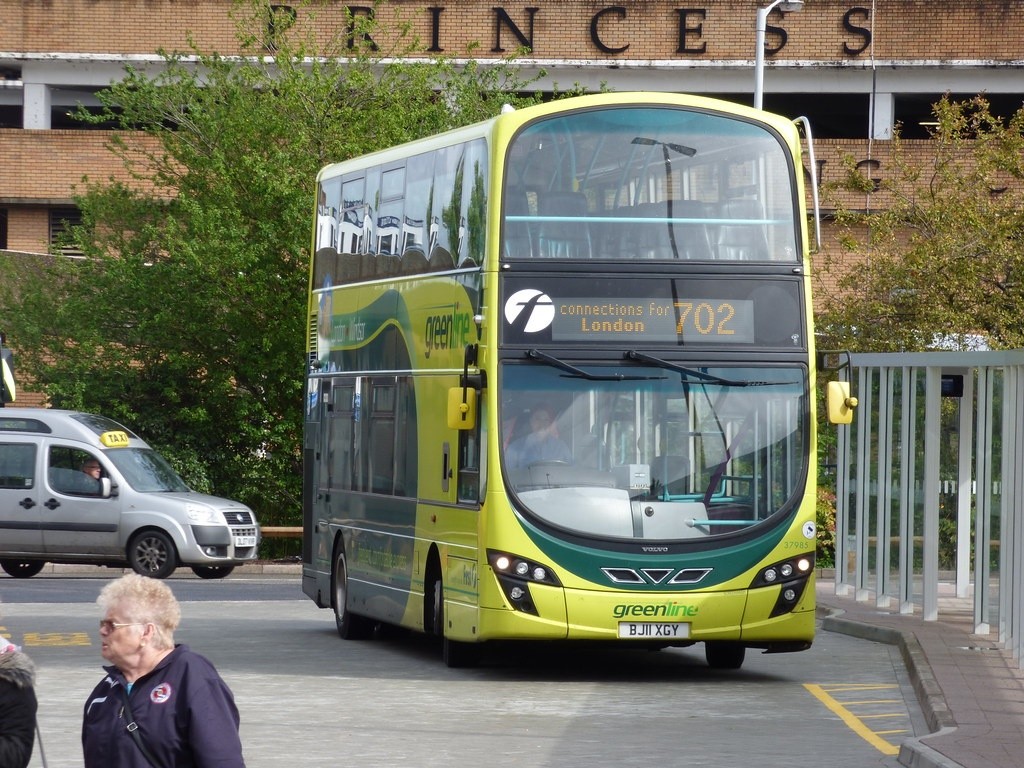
[75,455,101,493]
[0,633,39,768]
[504,407,572,469]
[81,574,246,768]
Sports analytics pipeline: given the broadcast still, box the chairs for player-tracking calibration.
[646,456,691,497]
[714,198,773,261]
[502,185,536,259]
[658,199,716,260]
[534,190,593,259]
[587,199,656,258]
[54,467,80,494]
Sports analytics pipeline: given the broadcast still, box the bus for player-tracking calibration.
[302,93,858,670]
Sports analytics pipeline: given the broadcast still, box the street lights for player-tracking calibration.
[755,1,804,111]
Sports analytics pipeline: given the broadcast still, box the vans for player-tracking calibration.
[0,408,261,579]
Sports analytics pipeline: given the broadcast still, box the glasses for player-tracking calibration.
[99,620,144,631]
